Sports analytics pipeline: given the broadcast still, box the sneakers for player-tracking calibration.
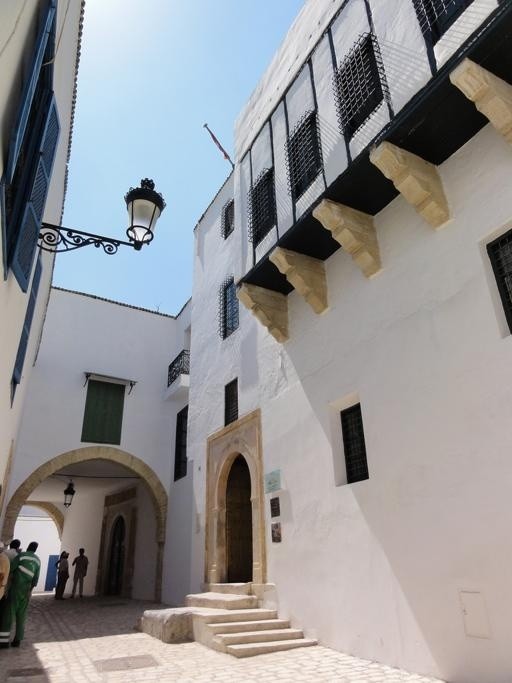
[0,640,20,649]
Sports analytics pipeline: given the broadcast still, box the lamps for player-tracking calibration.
[34,178,166,255]
[63,478,75,508]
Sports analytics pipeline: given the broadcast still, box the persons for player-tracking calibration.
[70,548,89,598]
[0,540,11,618]
[2,538,23,565]
[0,541,42,649]
[54,550,71,598]
